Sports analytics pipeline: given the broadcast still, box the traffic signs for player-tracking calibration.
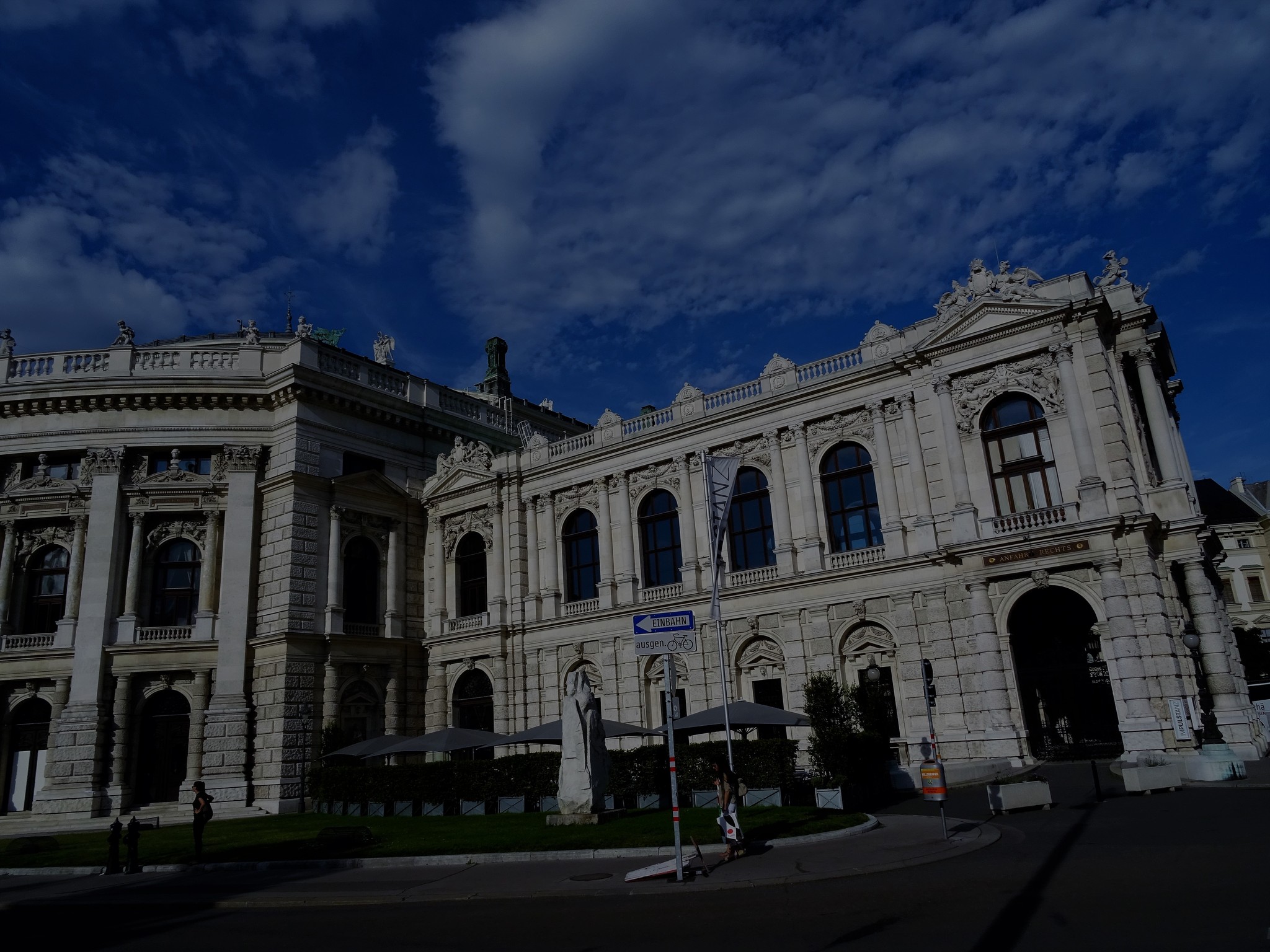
[632,610,695,636]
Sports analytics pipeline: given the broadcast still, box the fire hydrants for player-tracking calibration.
[124,816,141,874]
[105,817,122,875]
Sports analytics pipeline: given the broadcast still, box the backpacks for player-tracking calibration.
[738,778,748,797]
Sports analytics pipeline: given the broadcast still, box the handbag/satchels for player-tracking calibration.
[716,810,744,844]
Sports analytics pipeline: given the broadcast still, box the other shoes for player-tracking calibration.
[720,851,736,861]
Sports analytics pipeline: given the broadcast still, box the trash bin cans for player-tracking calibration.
[920,759,948,801]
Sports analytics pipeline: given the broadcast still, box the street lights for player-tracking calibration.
[1178,621,1248,781]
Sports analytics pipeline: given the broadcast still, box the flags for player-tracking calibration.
[706,455,740,623]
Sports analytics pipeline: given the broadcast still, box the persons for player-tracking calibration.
[191,781,215,849]
[711,760,746,861]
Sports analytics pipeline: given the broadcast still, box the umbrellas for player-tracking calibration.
[643,700,838,740]
[314,735,414,761]
[476,716,666,750]
[360,728,510,761]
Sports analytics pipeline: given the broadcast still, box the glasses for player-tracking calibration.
[712,764,717,768]
